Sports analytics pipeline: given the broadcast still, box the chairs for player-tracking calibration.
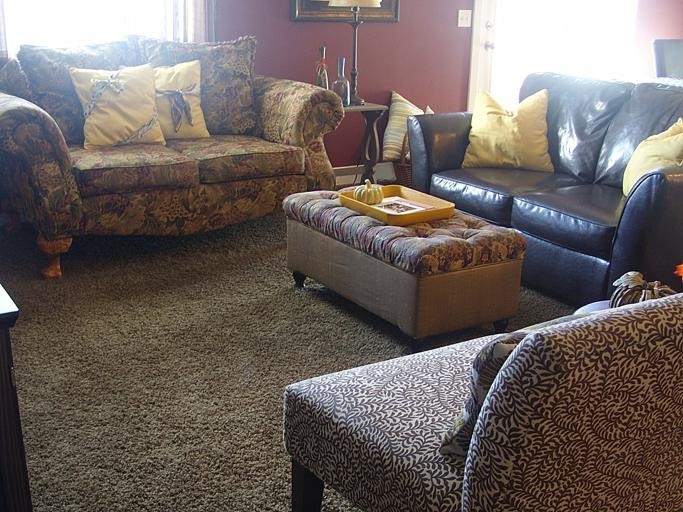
[284,294,683,512]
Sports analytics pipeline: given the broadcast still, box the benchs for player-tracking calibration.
[282,187,526,354]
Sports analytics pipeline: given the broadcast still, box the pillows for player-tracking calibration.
[461,72,683,197]
[382,91,425,162]
[0,35,257,149]
[438,332,541,457]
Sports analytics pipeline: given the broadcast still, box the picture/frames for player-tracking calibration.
[290,0,400,23]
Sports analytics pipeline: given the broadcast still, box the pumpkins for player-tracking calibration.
[352,178,384,205]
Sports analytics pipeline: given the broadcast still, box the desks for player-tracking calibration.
[0,284,33,512]
[343,102,388,185]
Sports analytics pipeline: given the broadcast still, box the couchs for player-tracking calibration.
[0,36,344,280]
[407,111,683,308]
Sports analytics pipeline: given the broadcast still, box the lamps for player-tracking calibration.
[328,0,381,106]
